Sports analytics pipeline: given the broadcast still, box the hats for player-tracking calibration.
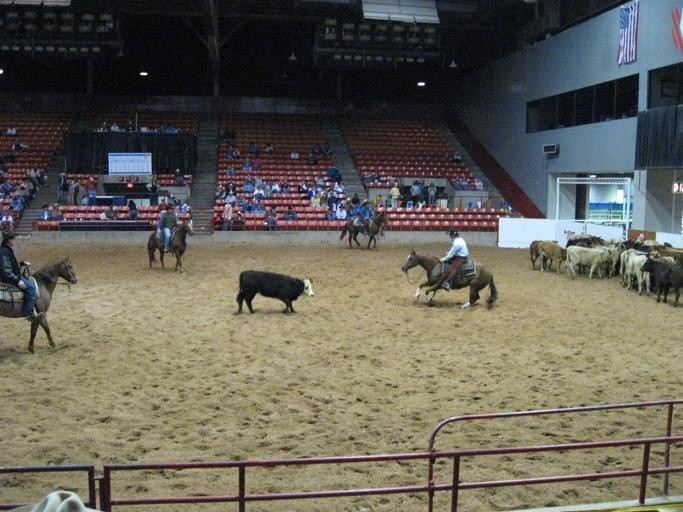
[447,229,458,235]
[2,227,16,241]
[165,205,175,209]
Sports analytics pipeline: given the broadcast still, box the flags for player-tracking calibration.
[617,1,639,65]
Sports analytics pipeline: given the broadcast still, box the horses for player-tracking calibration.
[0,254,77,355]
[338,210,387,250]
[400,250,497,309]
[148,221,193,274]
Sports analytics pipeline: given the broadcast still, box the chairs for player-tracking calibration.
[0,112,78,229]
[82,111,198,133]
[374,206,507,230]
[338,114,476,190]
[33,172,192,230]
[213,113,352,230]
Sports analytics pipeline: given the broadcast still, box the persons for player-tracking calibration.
[214,125,483,237]
[0,228,38,321]
[0,122,194,225]
[158,205,177,253]
[438,227,469,290]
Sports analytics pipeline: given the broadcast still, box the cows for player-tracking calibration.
[537,241,567,275]
[564,229,683,309]
[530,241,558,270]
[235,270,315,314]
